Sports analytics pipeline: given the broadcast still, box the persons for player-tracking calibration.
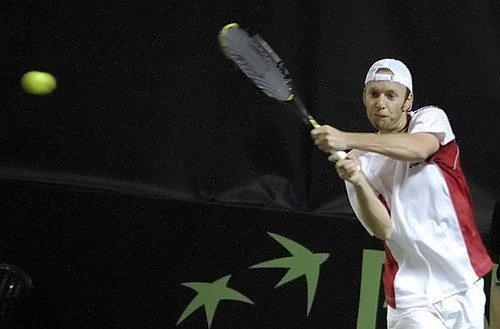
[310,58,494,329]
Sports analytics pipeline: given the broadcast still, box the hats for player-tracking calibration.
[364,57,413,92]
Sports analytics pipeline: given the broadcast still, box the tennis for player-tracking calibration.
[21,71,56,94]
